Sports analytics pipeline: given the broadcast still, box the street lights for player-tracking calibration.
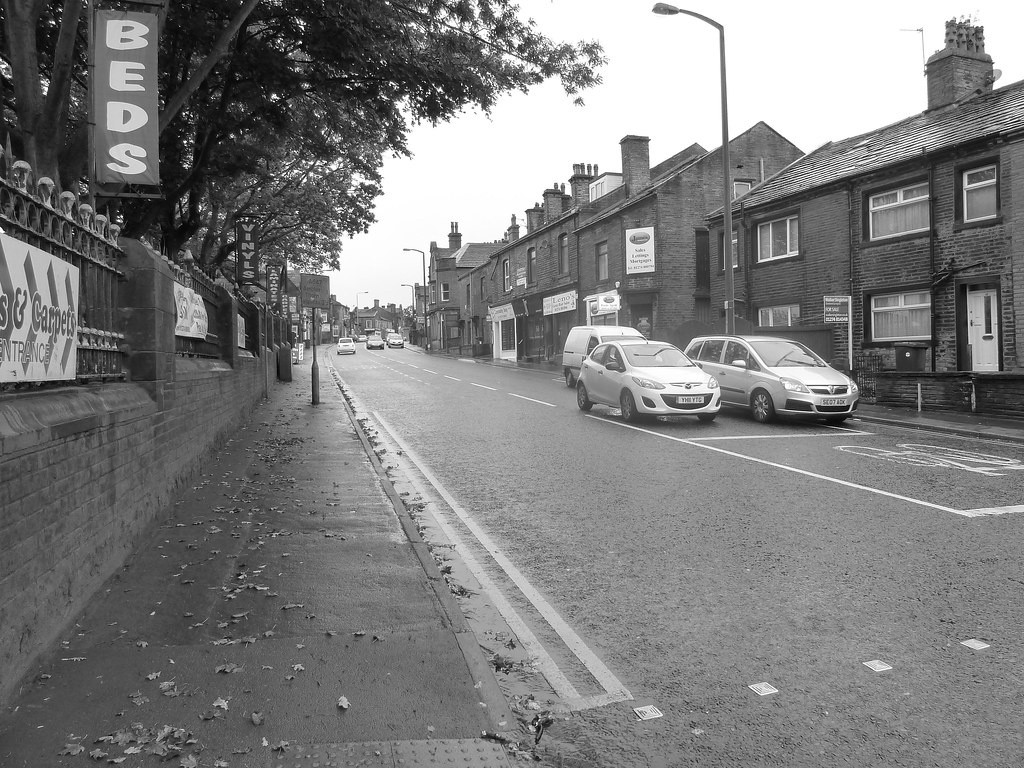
[402,248,428,353]
[651,0,735,340]
[399,283,415,346]
[356,290,369,331]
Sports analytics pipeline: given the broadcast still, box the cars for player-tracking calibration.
[684,335,861,425]
[337,337,357,354]
[575,340,722,423]
[366,336,384,349]
[347,335,357,343]
[383,327,399,344]
[388,335,405,349]
[357,335,366,341]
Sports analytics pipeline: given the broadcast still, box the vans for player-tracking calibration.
[562,325,663,389]
